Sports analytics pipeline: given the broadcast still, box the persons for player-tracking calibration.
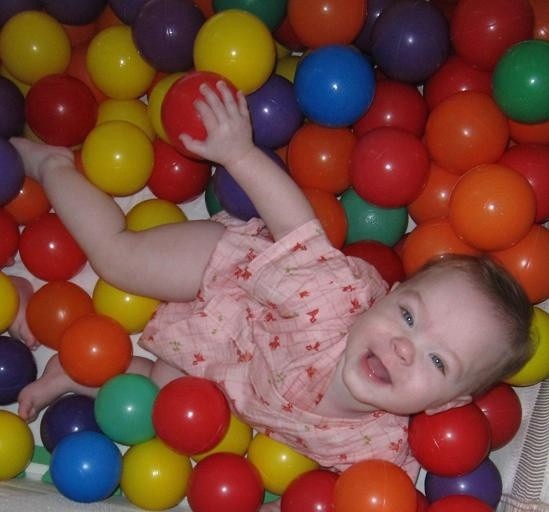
[8,80,538,512]
